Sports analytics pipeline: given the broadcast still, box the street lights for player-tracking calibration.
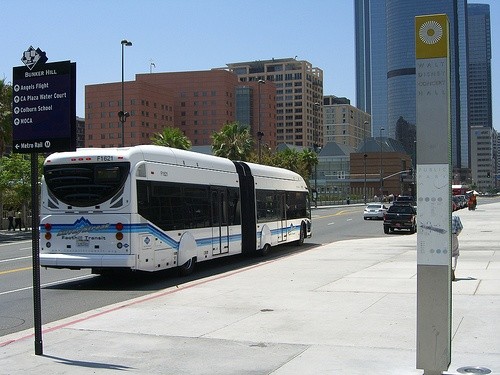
[379,127,385,196]
[414,140,417,170]
[118,39,133,146]
[256,79,266,164]
[313,102,320,149]
[363,120,369,205]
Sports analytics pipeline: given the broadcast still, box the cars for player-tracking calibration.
[451,195,470,212]
[363,204,388,220]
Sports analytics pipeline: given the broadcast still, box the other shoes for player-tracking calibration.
[451,269,455,281]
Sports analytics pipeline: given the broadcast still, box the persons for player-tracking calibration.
[8,207,15,231]
[14,208,22,231]
[452,202,463,280]
[468,194,477,210]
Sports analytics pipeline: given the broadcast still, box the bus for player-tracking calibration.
[39,144,313,277]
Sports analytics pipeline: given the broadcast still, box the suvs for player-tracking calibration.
[382,195,417,234]
